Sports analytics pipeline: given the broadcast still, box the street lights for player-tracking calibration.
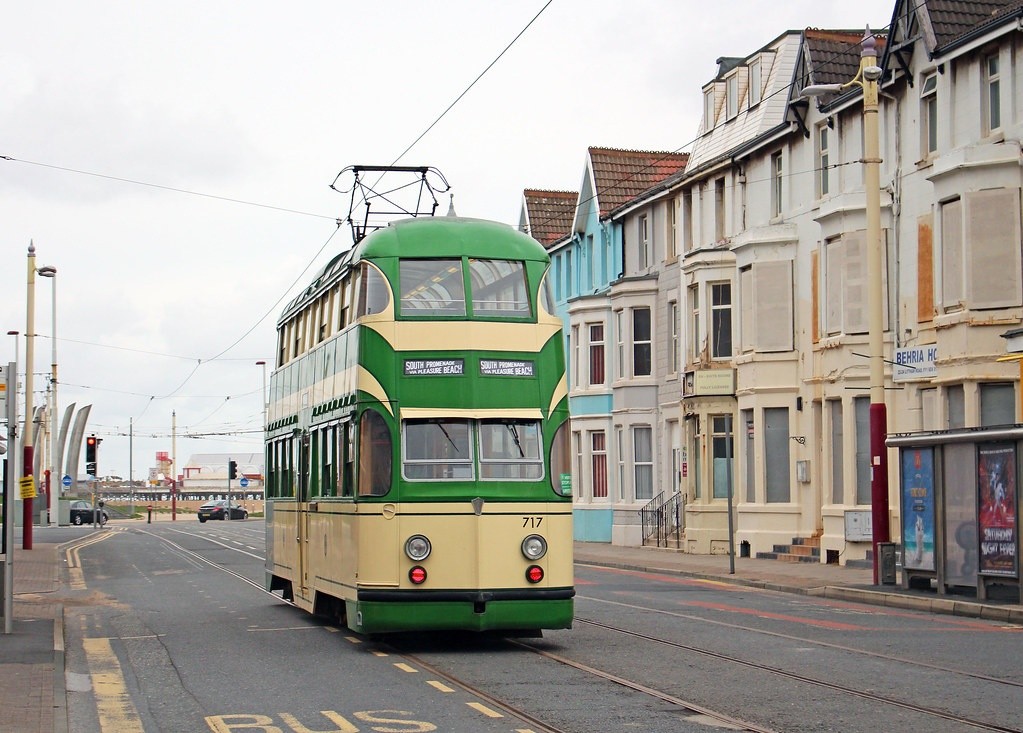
[37,266,58,529]
[5,330,40,637]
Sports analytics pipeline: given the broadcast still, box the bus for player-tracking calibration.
[262,163,576,641]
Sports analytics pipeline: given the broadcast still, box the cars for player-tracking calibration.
[198,500,249,523]
[47,500,109,525]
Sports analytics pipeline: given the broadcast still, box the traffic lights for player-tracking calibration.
[86,437,97,463]
[229,461,238,479]
